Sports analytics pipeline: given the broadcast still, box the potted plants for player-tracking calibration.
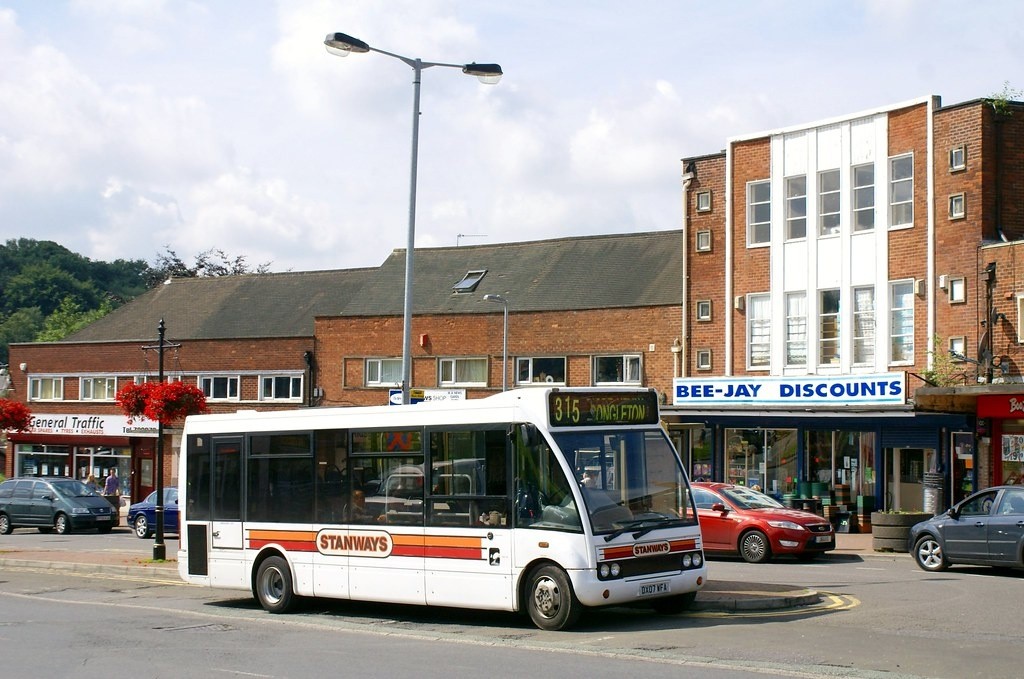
[870,505,934,553]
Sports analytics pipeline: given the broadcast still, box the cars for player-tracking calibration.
[584,454,614,477]
[648,483,836,564]
[907,485,1024,573]
[127,486,179,539]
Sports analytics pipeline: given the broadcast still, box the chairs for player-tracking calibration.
[1009,497,1024,513]
[192,463,469,526]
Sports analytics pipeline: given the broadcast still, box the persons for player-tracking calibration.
[342,490,386,523]
[751,485,761,492]
[104,468,120,495]
[697,477,704,482]
[580,471,597,488]
[83,474,103,495]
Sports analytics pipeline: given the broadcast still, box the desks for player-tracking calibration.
[790,498,824,517]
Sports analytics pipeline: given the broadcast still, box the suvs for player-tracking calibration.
[0,478,120,535]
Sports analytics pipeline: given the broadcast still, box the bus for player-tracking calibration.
[177,387,707,632]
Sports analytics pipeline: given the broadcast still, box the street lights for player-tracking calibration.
[484,295,508,392]
[324,32,503,405]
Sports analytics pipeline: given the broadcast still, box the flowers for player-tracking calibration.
[115,381,210,425]
[0,394,36,435]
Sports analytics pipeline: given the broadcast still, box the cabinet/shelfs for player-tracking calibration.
[692,442,756,488]
[960,467,973,494]
